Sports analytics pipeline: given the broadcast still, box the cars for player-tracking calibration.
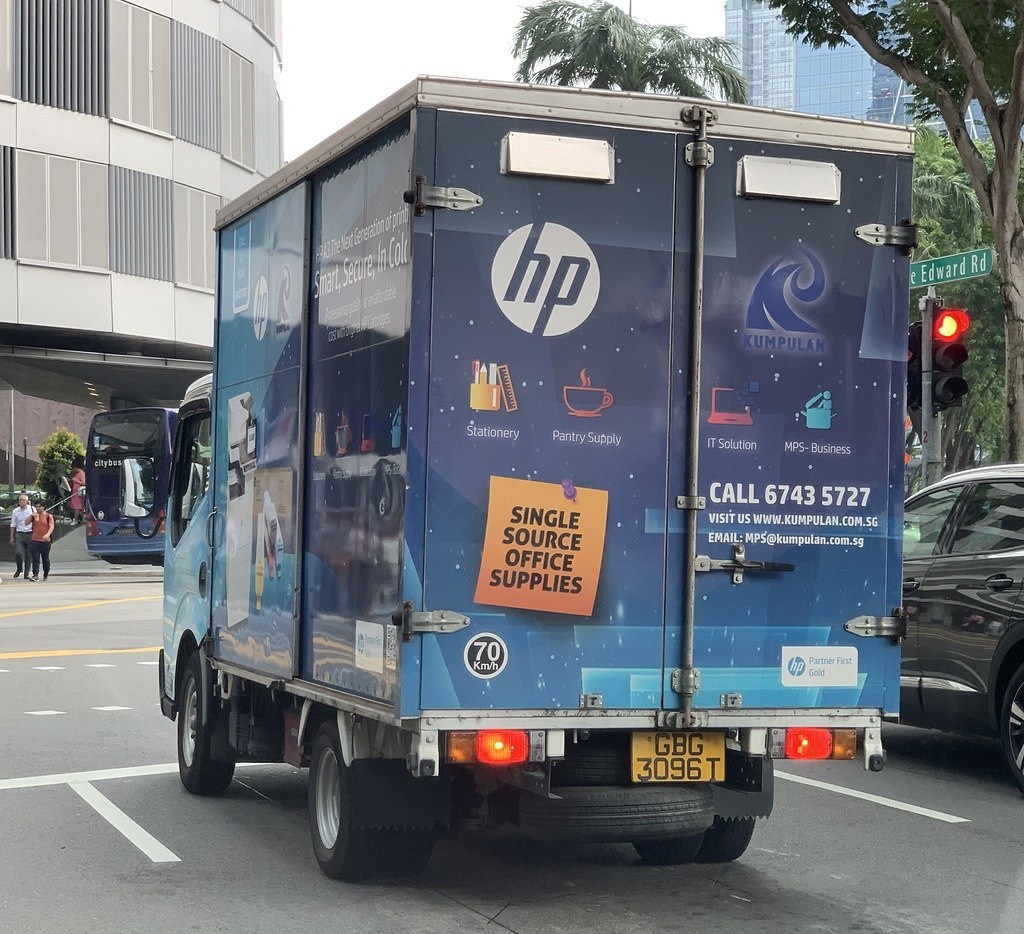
[882,464,1023,794]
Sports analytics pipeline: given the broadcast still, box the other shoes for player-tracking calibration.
[14,571,22,578]
[24,576,31,579]
[30,577,39,582]
[70,519,78,526]
[43,578,48,582]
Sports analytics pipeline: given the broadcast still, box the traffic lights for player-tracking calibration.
[929,305,970,408]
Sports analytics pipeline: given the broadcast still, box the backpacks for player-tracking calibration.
[32,512,54,543]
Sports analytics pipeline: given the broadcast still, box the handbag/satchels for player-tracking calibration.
[78,486,86,496]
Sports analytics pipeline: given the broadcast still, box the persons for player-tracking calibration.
[25,499,54,581]
[69,459,85,525]
[10,494,37,581]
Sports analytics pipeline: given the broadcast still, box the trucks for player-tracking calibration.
[159,74,914,882]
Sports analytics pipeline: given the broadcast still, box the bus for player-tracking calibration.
[86,408,182,567]
